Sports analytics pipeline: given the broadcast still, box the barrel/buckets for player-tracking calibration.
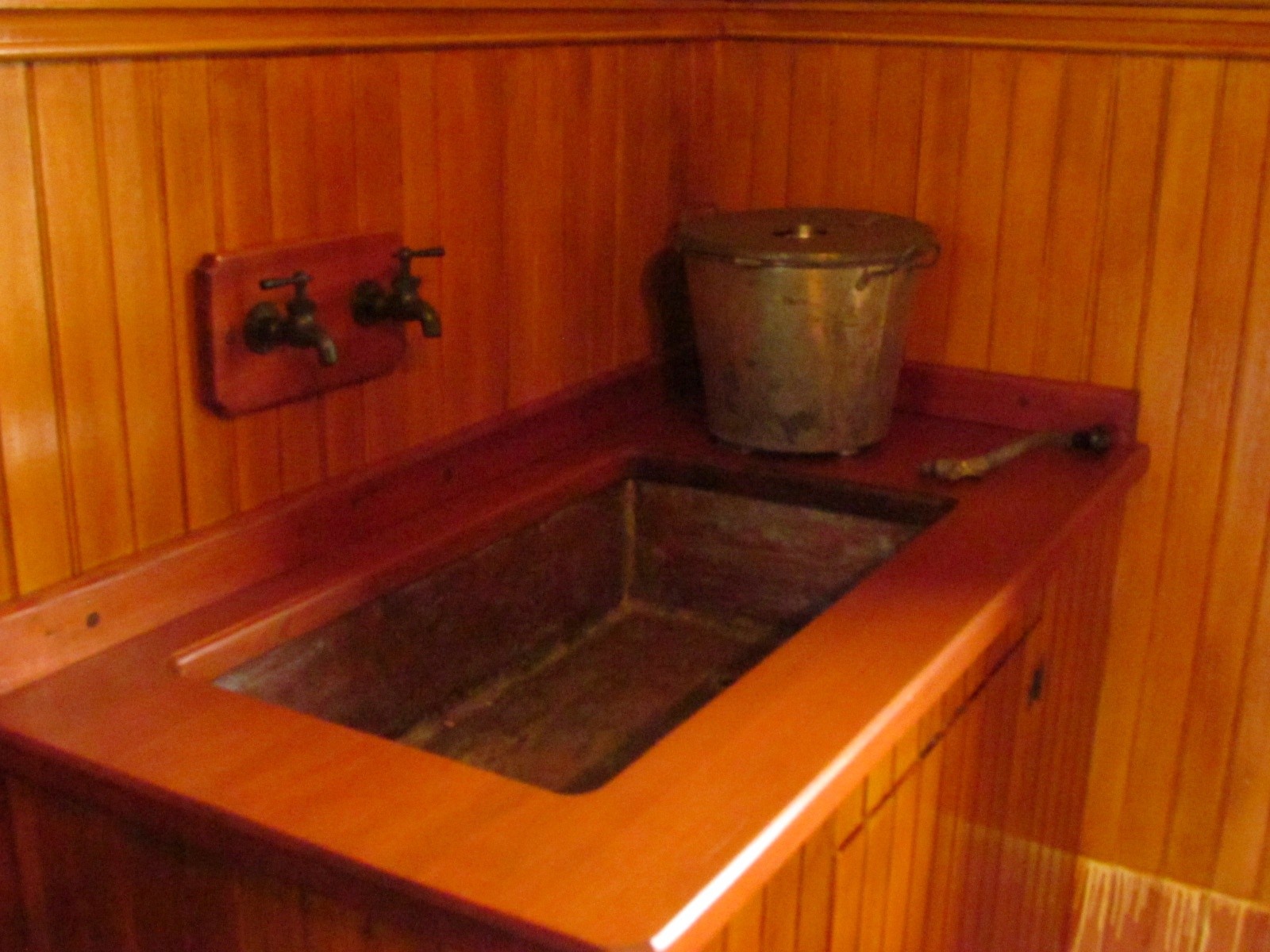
[671,206,942,456]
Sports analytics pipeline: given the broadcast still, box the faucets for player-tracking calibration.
[248,268,340,369]
[353,246,453,341]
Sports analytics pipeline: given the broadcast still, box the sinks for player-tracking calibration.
[168,444,966,803]
